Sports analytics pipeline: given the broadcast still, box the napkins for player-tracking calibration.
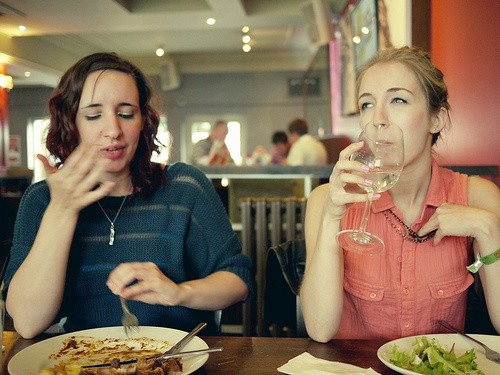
[276,351,382,375]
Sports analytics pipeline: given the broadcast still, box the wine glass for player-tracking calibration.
[335,119,405,256]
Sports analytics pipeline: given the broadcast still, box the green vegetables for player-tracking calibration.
[389,337,485,375]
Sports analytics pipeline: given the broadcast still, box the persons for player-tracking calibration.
[2,52,258,344]
[264,131,291,170]
[187,120,235,189]
[276,118,327,200]
[300,45,500,343]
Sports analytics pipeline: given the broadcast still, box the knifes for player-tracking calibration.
[82,346,224,369]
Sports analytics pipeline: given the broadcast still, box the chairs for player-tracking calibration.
[1,223,244,333]
[290,239,484,339]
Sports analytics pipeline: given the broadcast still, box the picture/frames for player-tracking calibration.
[349,0,379,80]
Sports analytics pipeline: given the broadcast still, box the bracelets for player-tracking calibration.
[466,246,500,273]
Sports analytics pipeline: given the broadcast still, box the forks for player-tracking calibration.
[120,296,140,337]
[439,319,500,365]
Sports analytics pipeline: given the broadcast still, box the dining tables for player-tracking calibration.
[0,330,408,375]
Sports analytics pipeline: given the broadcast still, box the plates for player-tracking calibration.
[8,325,210,375]
[377,333,500,375]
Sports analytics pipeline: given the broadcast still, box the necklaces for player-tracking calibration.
[89,175,135,245]
[379,204,440,244]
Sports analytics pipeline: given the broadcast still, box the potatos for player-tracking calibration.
[40,363,86,375]
